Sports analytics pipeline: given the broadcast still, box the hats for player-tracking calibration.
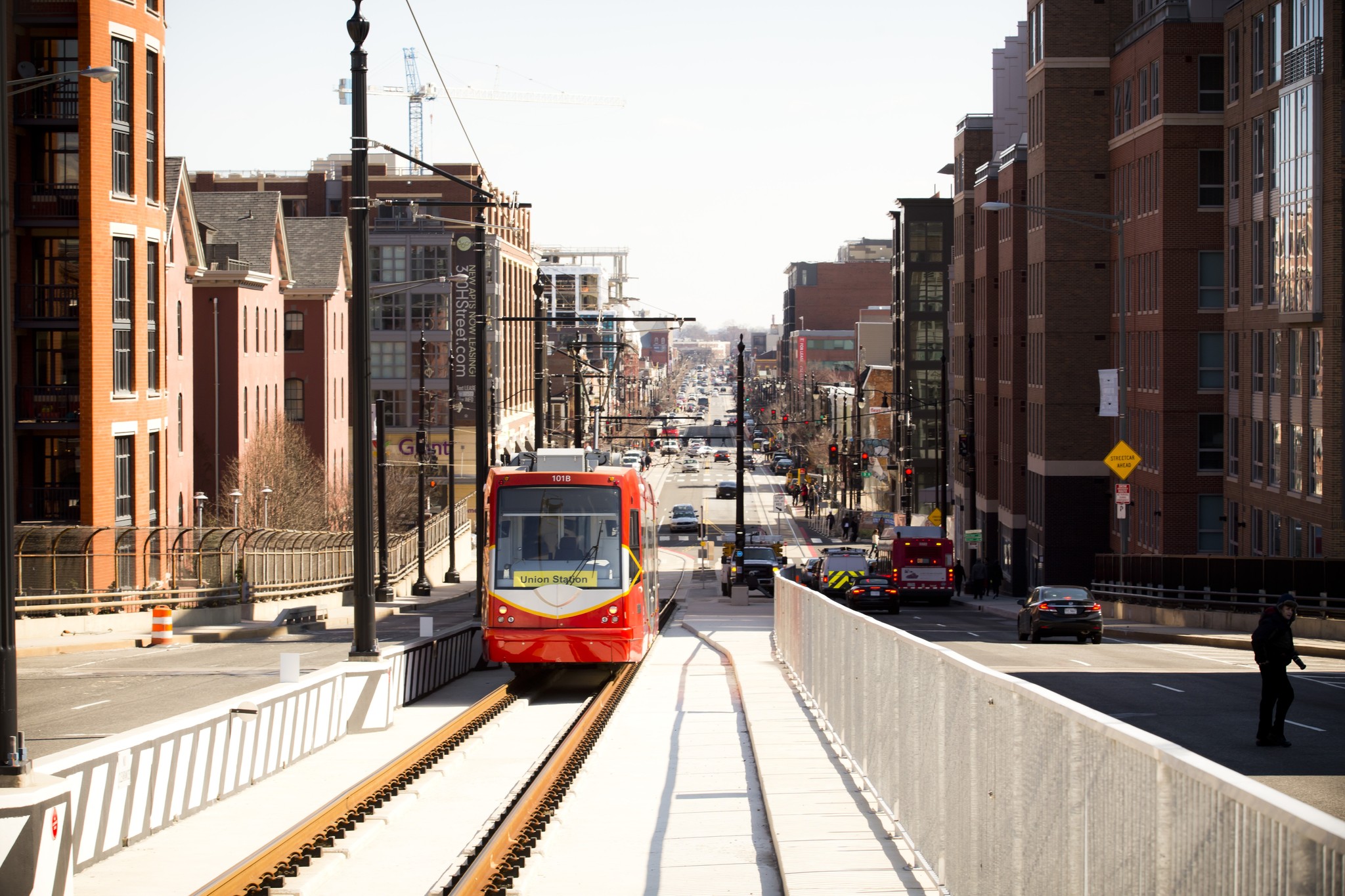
[1277,593,1297,621]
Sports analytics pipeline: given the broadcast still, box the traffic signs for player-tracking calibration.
[774,495,784,513]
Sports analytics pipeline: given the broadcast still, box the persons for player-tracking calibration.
[676,406,685,412]
[826,512,835,536]
[721,438,727,447]
[792,482,827,519]
[850,516,858,543]
[1251,593,1298,748]
[868,529,880,559]
[764,446,773,461]
[649,441,654,452]
[705,438,711,447]
[877,517,885,535]
[954,560,966,597]
[841,513,850,542]
[971,558,1003,600]
[639,454,652,472]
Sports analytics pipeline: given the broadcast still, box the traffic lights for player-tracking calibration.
[430,481,437,488]
[771,410,776,421]
[743,387,745,392]
[906,468,912,489]
[746,395,750,402]
[828,444,840,466]
[862,453,867,470]
[761,402,765,412]
[430,455,438,472]
[782,415,789,429]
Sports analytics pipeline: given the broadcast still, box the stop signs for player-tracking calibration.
[784,542,788,546]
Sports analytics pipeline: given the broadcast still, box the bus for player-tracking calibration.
[875,525,956,607]
[477,341,660,682]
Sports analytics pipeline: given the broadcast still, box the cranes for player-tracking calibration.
[339,46,435,177]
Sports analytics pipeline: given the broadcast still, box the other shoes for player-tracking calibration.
[993,594,996,599]
[996,597,998,600]
[1268,723,1292,747]
[1256,737,1280,746]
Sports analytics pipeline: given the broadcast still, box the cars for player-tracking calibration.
[621,360,801,533]
[1016,585,1103,645]
[846,576,900,615]
[800,547,869,599]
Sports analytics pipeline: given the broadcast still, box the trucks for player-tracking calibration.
[718,534,788,599]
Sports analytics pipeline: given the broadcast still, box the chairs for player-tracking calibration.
[554,538,582,560]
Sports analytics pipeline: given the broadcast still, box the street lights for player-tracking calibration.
[880,392,939,510]
[982,201,1129,618]
[194,486,274,585]
[349,271,473,661]
[814,382,863,524]
[856,388,912,526]
